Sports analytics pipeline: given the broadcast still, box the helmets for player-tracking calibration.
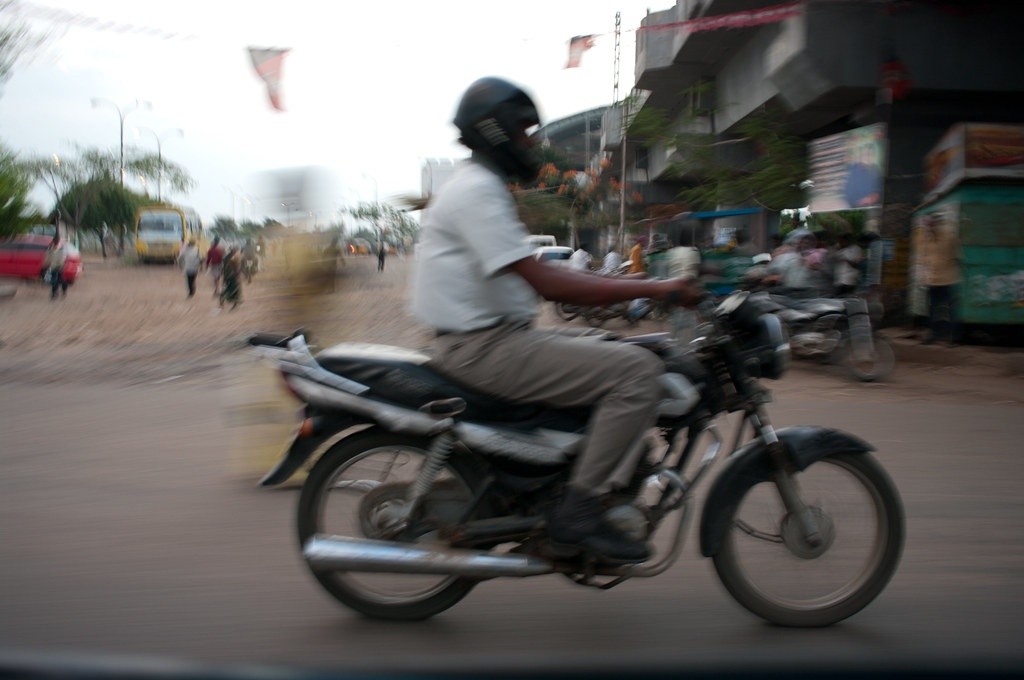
[453,76,546,188]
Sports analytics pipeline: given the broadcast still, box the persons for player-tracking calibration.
[571,221,876,316]
[223,237,405,310]
[917,212,964,348]
[47,235,70,300]
[206,234,227,297]
[407,76,706,564]
[845,141,883,209]
[177,238,204,298]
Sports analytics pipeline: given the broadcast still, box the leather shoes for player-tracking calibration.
[538,488,654,561]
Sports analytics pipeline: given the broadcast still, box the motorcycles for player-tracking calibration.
[242,257,908,626]
[567,218,898,383]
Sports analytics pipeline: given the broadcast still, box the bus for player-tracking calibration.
[135,202,203,266]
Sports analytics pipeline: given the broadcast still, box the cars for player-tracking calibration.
[0,234,82,285]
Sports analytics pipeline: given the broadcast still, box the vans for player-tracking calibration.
[524,235,558,251]
[535,245,570,265]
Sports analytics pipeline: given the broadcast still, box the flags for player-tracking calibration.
[638,4,802,34]
[249,48,287,112]
[565,36,593,70]
